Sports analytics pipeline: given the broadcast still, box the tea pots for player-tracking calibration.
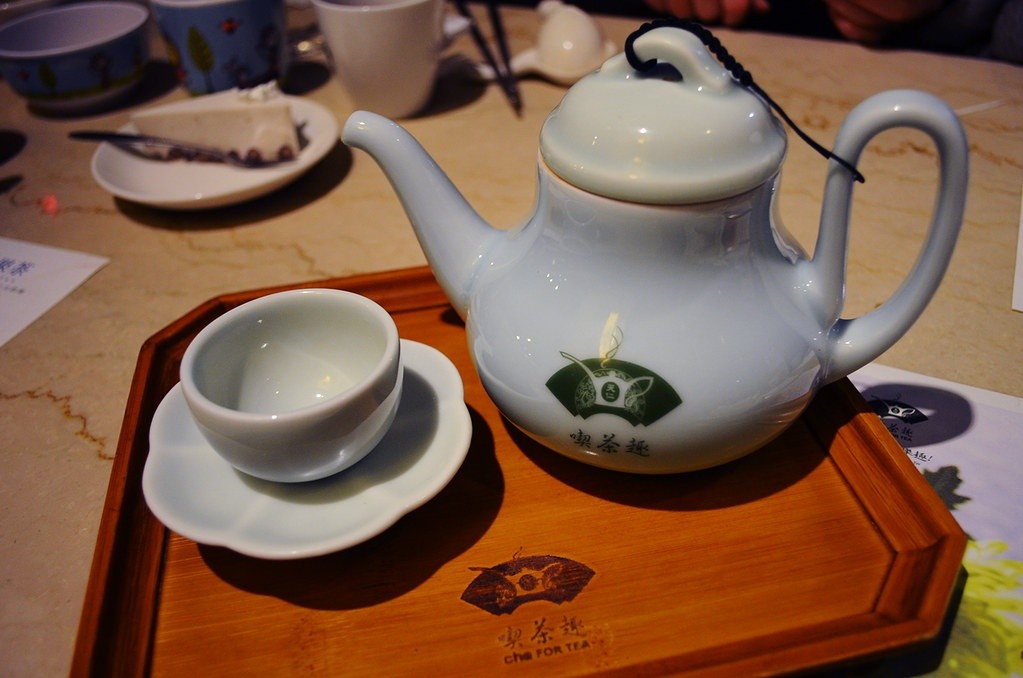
[338,19,970,476]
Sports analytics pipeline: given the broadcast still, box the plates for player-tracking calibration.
[141,336,473,562]
[90,91,340,212]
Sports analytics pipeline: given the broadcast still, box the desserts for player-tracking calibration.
[130,79,300,164]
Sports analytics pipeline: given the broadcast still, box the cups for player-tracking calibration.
[146,0,290,98]
[310,0,444,119]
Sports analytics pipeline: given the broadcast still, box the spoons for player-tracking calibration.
[67,130,296,168]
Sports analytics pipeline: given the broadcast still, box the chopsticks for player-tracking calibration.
[451,0,521,112]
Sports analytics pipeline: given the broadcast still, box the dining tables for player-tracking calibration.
[0,0,1023,678]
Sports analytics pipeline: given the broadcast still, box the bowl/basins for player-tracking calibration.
[179,290,403,483]
[0,2,149,119]
